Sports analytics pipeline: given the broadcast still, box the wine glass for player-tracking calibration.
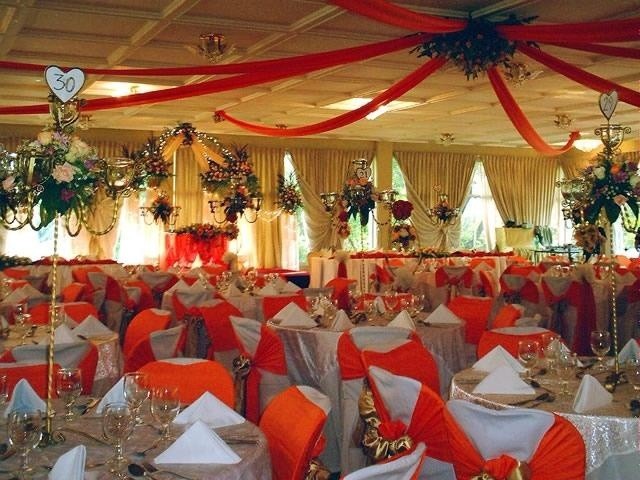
[195,269,280,292]
[0,277,65,345]
[130,260,184,280]
[0,369,180,480]
[549,263,610,279]
[307,289,426,328]
[518,329,639,408]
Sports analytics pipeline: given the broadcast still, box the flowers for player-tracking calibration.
[390,224,418,250]
[127,129,181,228]
[389,199,415,222]
[427,192,459,226]
[556,148,640,262]
[318,152,402,225]
[1,98,136,237]
[274,166,308,215]
[201,140,268,226]
[176,123,199,147]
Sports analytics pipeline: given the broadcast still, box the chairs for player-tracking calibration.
[1,264,640,478]
